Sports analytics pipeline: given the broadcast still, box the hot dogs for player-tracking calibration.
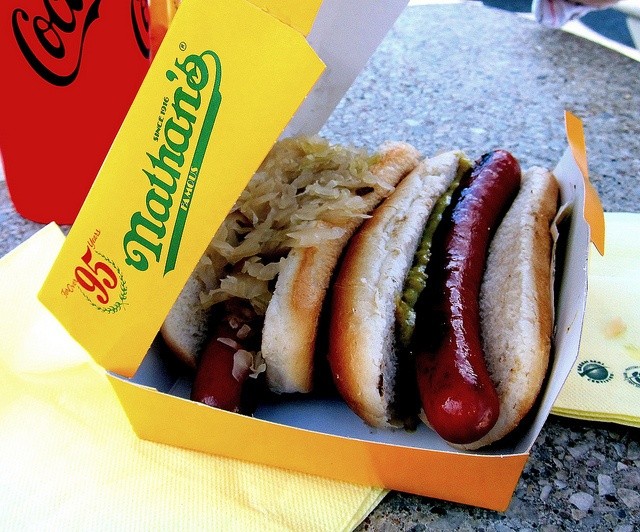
[327,146,563,457]
[160,130,420,427]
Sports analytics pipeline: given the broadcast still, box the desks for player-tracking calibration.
[0,1,639,532]
